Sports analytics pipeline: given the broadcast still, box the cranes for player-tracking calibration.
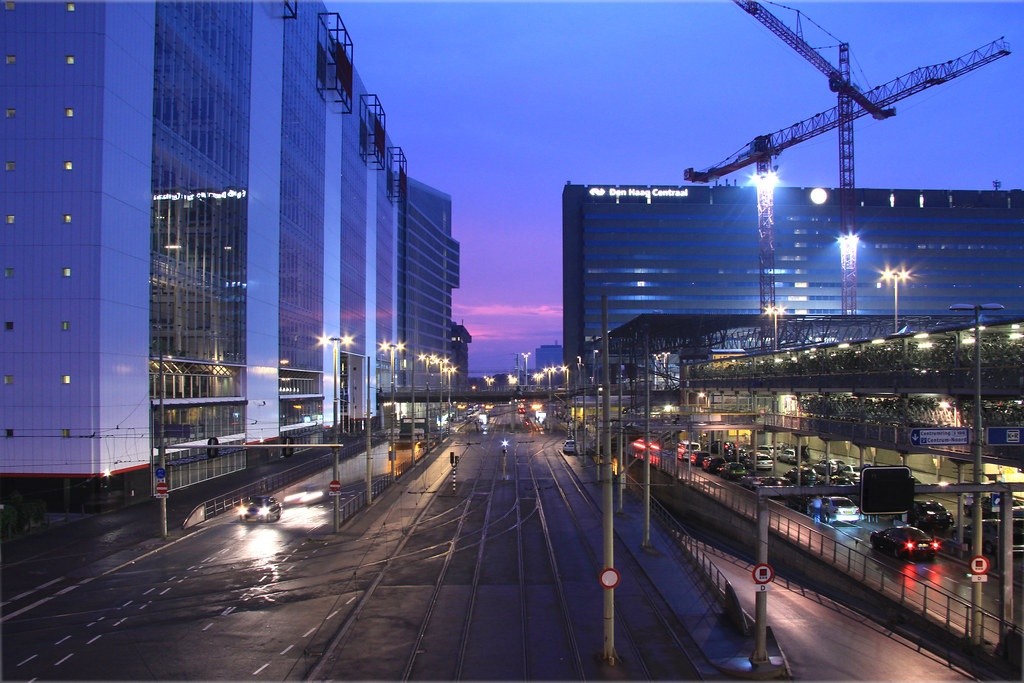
[731,0,896,325]
[681,29,1014,368]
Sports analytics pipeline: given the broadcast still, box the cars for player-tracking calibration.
[674,437,1024,563]
[563,440,576,455]
[240,496,281,524]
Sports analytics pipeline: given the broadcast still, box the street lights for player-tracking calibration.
[419,353,456,449]
[317,332,353,503]
[379,341,407,460]
[521,351,531,388]
[487,363,570,422]
